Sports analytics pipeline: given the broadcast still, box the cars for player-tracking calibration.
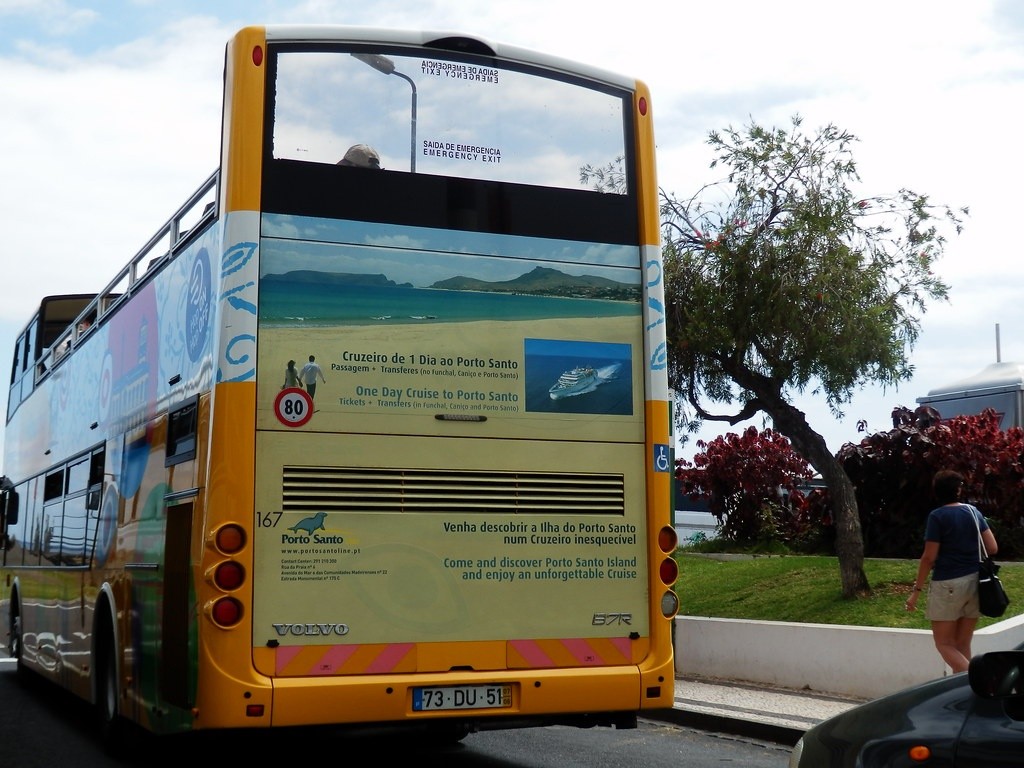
[784,638,1024,768]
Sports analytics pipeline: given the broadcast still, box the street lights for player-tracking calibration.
[348,52,419,173]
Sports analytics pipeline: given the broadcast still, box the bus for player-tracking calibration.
[0,28,681,739]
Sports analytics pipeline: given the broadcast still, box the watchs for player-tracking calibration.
[912,584,921,593]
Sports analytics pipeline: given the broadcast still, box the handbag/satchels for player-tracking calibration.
[978,558,1011,618]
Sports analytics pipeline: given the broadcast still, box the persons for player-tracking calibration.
[335,145,386,171]
[902,471,996,675]
[298,355,326,401]
[279,360,304,390]
[77,309,97,336]
